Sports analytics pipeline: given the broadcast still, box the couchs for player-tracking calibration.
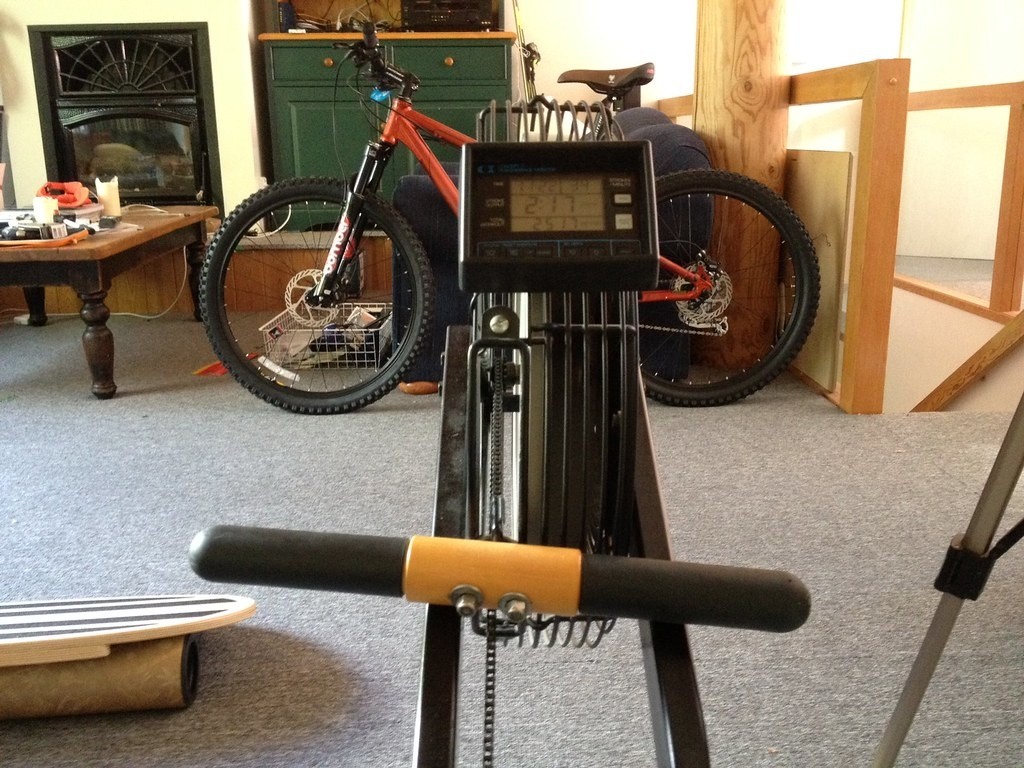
[393,105,716,393]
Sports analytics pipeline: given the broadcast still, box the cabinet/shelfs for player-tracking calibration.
[259,32,519,233]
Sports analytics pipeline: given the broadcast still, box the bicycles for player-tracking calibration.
[195,19,821,416]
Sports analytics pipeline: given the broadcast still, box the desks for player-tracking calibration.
[1,205,219,401]
